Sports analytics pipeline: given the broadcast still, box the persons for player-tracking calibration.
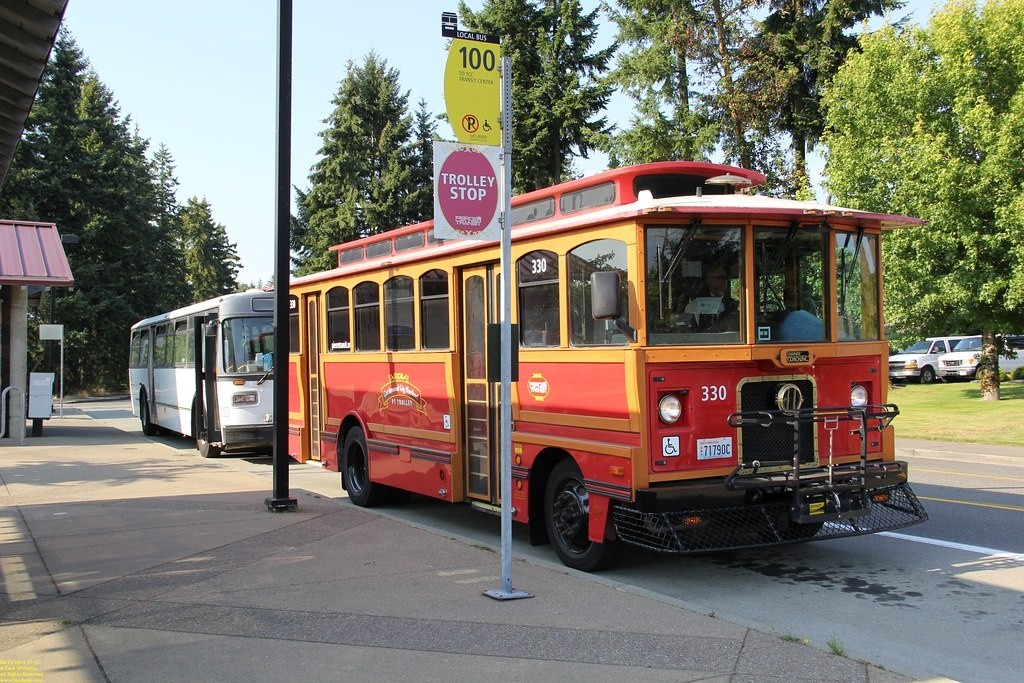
[801,282,818,317]
[681,260,741,328]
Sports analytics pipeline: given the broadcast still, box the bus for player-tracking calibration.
[128,288,275,458]
[287,160,933,574]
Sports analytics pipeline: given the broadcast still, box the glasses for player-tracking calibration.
[709,275,728,280]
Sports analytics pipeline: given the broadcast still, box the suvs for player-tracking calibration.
[936,333,1024,384]
[888,335,966,383]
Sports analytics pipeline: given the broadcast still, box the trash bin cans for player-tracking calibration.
[27,372,55,436]
